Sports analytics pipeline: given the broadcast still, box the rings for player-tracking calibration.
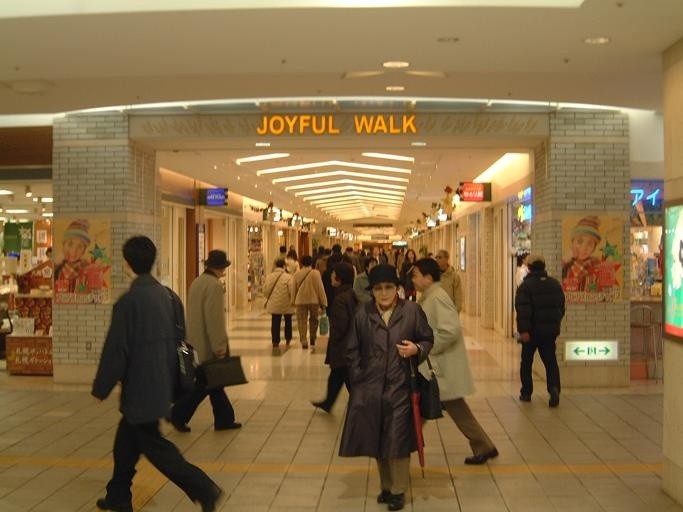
[403,353,406,359]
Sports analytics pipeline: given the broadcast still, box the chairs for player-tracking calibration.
[629,304,660,385]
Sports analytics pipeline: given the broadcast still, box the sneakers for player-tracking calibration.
[272,342,293,351]
[96,492,134,512]
[312,402,330,413]
[549,385,560,408]
[165,413,191,433]
[519,393,532,401]
[302,342,315,354]
[197,481,223,512]
[213,419,243,431]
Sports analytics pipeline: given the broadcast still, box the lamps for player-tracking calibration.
[411,196,458,237]
[262,201,316,233]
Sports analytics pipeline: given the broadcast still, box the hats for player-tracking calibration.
[364,263,400,292]
[62,217,92,249]
[569,214,602,243]
[202,249,231,269]
[526,254,545,266]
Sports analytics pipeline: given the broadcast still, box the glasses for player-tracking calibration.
[435,255,445,260]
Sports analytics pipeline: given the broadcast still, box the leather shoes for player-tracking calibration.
[464,445,498,466]
[376,488,392,504]
[389,493,404,511]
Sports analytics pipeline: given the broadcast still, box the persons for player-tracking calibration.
[562,212,612,293]
[87,232,224,511]
[162,247,247,433]
[408,257,500,466]
[260,244,464,356]
[511,248,531,344]
[54,218,101,294]
[305,257,361,415]
[333,261,436,511]
[513,253,566,404]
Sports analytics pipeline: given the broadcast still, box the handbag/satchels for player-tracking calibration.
[413,341,444,421]
[201,355,248,390]
[320,305,330,335]
